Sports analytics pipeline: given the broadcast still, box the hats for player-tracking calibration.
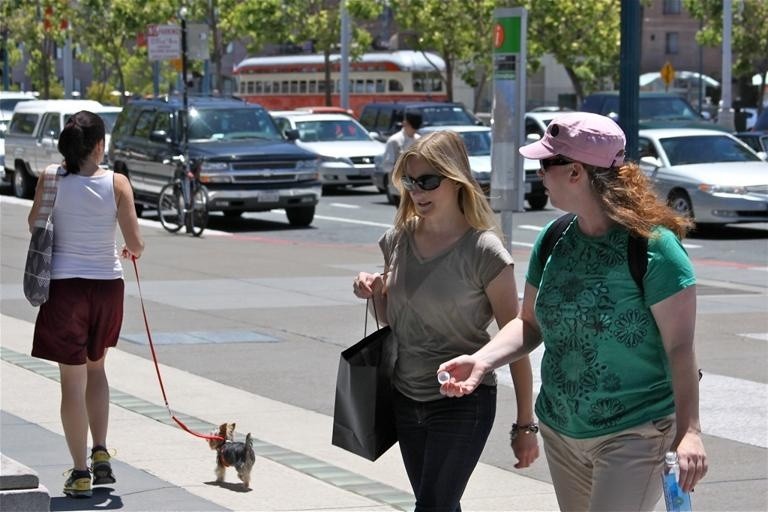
[519,112,626,169]
[405,110,423,129]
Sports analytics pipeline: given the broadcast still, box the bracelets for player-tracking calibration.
[511,422,539,435]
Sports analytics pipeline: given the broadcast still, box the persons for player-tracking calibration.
[437,110,709,512]
[383,108,424,211]
[26,110,144,497]
[352,127,540,512]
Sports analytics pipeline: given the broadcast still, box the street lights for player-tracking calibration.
[180,4,192,236]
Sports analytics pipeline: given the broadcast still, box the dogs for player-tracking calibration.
[206,422,256,489]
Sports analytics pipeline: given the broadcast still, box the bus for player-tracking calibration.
[233,51,448,121]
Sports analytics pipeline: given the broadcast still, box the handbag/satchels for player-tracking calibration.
[332,327,398,461]
[24,221,53,306]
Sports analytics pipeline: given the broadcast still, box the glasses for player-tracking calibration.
[400,174,448,191]
[541,157,573,172]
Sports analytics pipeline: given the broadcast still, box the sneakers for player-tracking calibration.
[88,445,117,485]
[63,468,93,498]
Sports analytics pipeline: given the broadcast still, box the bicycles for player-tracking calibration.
[155,154,212,237]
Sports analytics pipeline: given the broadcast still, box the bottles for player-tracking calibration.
[660,451,692,512]
